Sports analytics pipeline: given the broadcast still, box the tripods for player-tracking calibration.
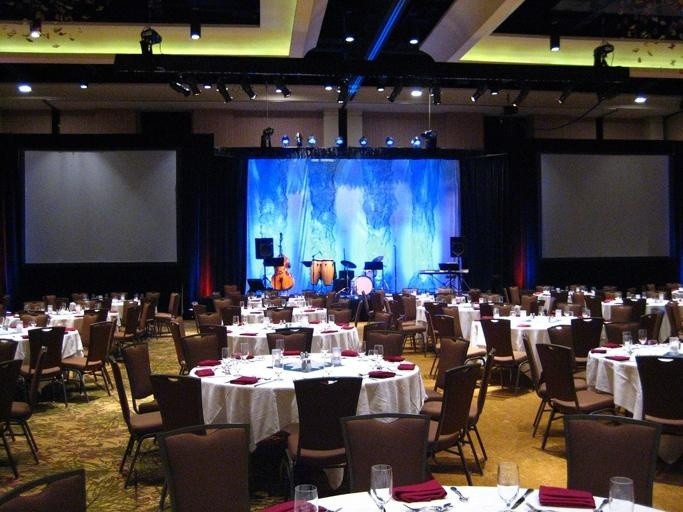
[445,257,472,292]
[335,264,357,296]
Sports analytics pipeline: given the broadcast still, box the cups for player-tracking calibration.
[332,347,341,366]
[272,349,281,368]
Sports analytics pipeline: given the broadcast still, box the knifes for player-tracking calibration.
[512,489,535,510]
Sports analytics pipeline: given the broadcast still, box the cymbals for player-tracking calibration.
[341,261,356,268]
[371,256,383,268]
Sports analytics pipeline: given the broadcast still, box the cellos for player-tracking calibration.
[272,232,294,290]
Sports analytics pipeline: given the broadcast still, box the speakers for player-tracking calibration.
[255,238,273,260]
[450,236,466,259]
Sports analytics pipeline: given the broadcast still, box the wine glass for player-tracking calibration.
[609,476,634,512]
[294,484,319,512]
[232,291,336,330]
[374,345,383,369]
[637,329,648,344]
[497,462,519,512]
[241,342,250,364]
[359,341,367,360]
[276,339,284,361]
[370,464,393,512]
[669,337,680,354]
[232,353,243,377]
[622,331,633,351]
[410,285,683,329]
[0,291,145,338]
[222,347,231,375]
[648,340,658,356]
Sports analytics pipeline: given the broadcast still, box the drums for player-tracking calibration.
[311,261,321,284]
[350,276,373,296]
[321,260,334,286]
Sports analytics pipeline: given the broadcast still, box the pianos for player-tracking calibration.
[420,268,467,274]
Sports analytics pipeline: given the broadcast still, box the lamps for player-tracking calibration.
[80,78,90,89]
[29,17,42,37]
[550,34,560,52]
[189,22,203,39]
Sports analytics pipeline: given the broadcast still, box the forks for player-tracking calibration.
[450,486,469,501]
[593,498,611,512]
